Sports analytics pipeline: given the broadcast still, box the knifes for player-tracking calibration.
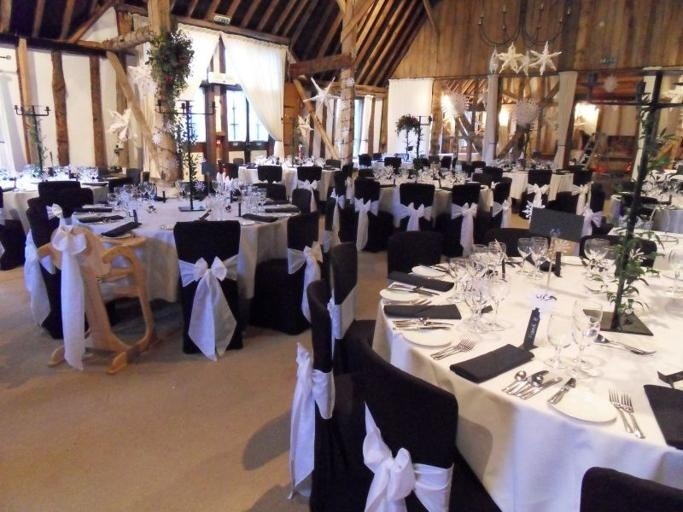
[397,319,452,328]
[516,370,547,395]
[522,378,559,399]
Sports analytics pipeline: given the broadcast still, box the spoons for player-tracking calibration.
[501,370,526,394]
[593,333,650,355]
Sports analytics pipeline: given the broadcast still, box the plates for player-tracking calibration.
[402,328,450,347]
[548,391,618,425]
[380,288,420,302]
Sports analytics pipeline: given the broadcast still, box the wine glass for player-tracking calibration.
[583,235,607,275]
[667,247,683,296]
[113,181,157,219]
[517,238,533,273]
[528,238,548,281]
[546,314,576,369]
[572,299,601,369]
[448,257,468,304]
[489,243,505,279]
[207,181,259,213]
[464,274,508,333]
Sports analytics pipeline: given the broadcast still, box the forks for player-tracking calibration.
[621,396,645,439]
[430,336,469,358]
[608,390,632,433]
[433,339,477,361]
